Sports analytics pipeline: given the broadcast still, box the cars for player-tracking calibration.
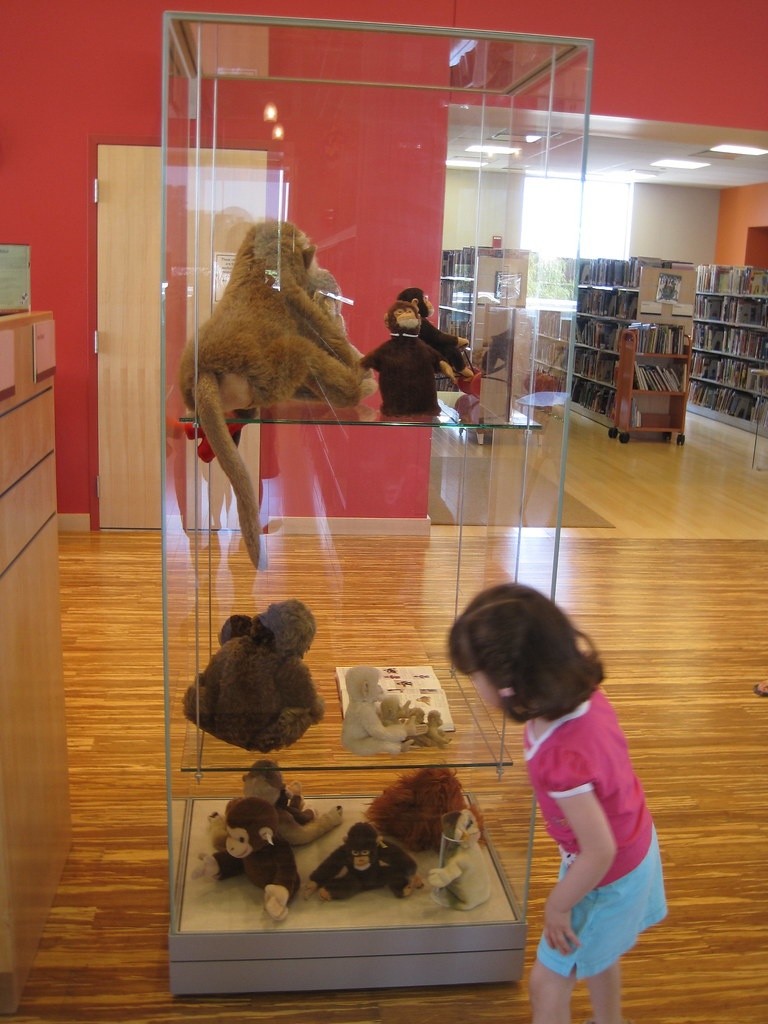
[452,292,500,304]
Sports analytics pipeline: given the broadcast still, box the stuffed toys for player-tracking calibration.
[208,759,343,850]
[183,599,323,754]
[429,809,490,909]
[481,329,510,374]
[181,220,376,571]
[302,822,424,902]
[367,766,483,853]
[203,796,300,920]
[341,666,452,756]
[360,288,474,416]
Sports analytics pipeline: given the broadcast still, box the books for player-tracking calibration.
[536,258,684,429]
[437,246,496,391]
[689,264,768,427]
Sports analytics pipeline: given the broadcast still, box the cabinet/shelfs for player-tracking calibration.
[676,264,767,439]
[157,6,594,997]
[536,255,699,442]
[434,247,530,442]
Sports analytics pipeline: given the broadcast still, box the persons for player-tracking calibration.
[449,583,667,1024]
